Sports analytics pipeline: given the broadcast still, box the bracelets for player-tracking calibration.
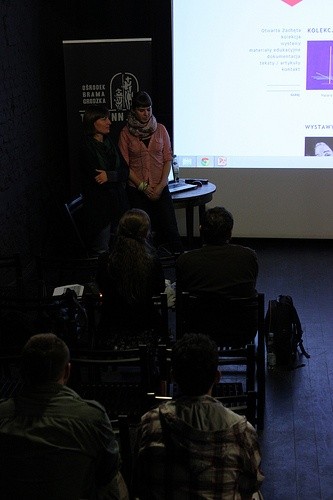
[138,181,148,191]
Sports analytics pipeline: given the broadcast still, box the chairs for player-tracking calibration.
[0,290,178,499]
[174,292,267,416]
[64,193,98,260]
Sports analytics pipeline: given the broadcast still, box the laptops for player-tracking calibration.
[167,154,198,194]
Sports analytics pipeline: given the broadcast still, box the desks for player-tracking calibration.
[170,178,216,245]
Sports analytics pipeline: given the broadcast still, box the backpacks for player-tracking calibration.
[265,295,310,377]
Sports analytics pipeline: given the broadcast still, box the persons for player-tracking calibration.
[176,207,260,348]
[120,92,182,256]
[82,106,127,255]
[126,331,267,500]
[0,327,125,500]
[82,211,168,348]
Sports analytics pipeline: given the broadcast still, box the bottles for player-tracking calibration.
[173,155,179,183]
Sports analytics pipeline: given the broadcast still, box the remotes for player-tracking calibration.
[185,179,209,184]
